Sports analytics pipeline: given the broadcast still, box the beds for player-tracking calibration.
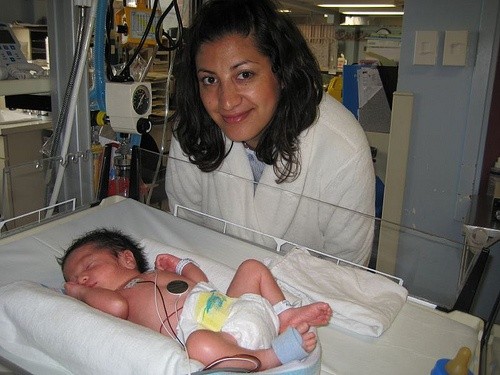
[0,142,500,375]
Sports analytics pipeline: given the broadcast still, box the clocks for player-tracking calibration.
[376,28,392,35]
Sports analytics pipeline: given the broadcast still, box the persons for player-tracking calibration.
[56,228,332,371]
[165,0,376,272]
